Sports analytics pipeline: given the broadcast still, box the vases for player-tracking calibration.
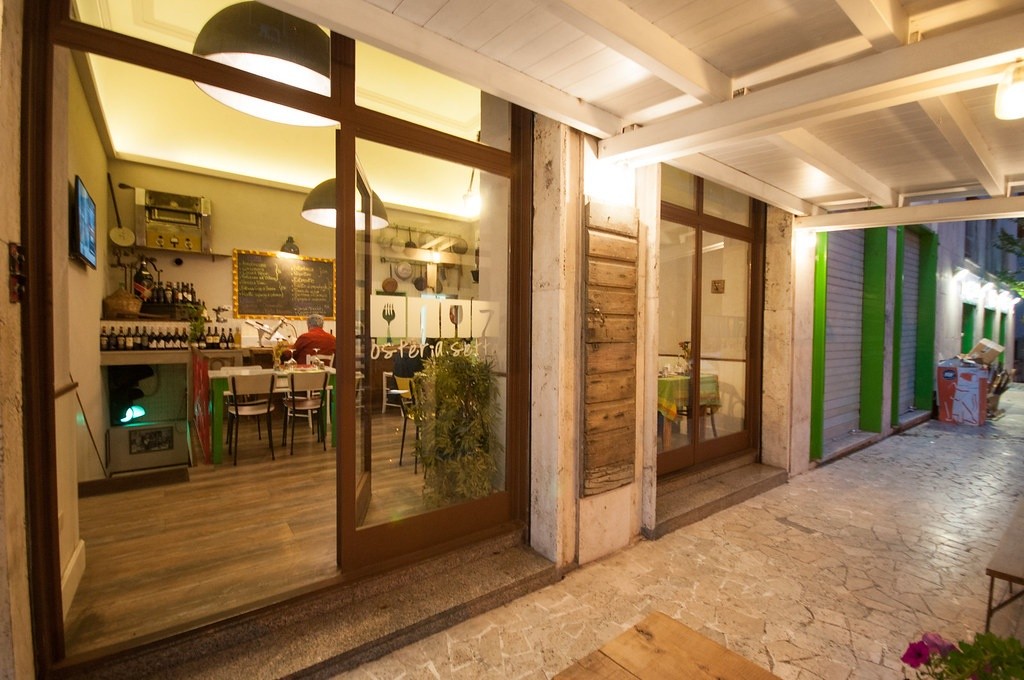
[684,364,692,376]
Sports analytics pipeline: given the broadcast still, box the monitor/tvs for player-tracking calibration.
[73,174,97,269]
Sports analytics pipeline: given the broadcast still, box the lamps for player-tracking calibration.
[192,1,340,126]
[280,237,299,254]
[993,60,1024,121]
[299,178,389,230]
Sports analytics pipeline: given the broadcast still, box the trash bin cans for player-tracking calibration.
[935,337,1018,427]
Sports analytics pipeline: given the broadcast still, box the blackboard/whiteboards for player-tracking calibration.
[231,247,336,319]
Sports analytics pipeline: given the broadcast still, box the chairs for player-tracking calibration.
[219,354,429,476]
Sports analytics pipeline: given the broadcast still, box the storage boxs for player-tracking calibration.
[968,338,1005,364]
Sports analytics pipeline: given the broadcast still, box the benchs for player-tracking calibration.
[984,503,1024,632]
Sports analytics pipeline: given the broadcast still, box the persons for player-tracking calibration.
[294,314,336,364]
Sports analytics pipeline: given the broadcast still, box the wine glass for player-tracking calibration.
[287,349,297,371]
[312,349,320,370]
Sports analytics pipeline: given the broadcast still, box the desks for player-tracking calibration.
[658,375,720,451]
[546,612,784,680]
[208,365,336,463]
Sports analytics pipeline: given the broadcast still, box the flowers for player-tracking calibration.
[900,628,1024,680]
[677,339,692,362]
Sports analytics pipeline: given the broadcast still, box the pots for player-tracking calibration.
[377,225,468,294]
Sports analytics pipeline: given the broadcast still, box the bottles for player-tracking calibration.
[938,353,944,366]
[134,257,153,303]
[152,282,196,304]
[192,327,242,349]
[101,326,189,350]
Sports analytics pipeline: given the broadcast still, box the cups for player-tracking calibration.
[662,367,668,378]
